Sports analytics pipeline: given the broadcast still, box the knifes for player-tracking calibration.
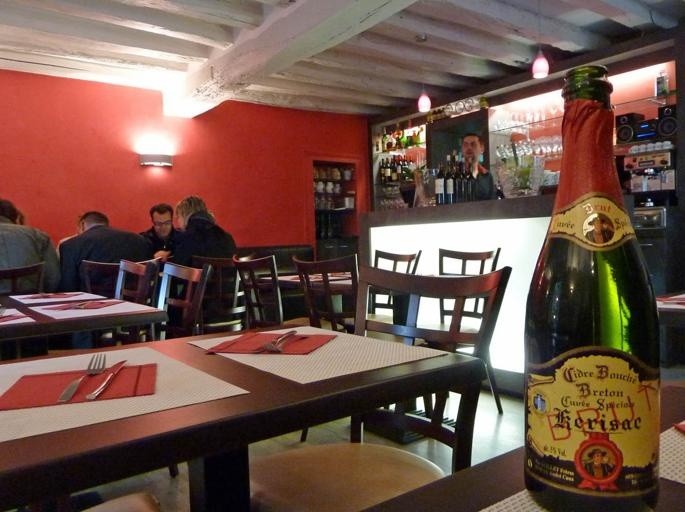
[247,329,297,353]
[86,358,130,401]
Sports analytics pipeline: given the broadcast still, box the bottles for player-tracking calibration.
[644,198,653,208]
[312,163,354,240]
[655,66,668,96]
[424,98,480,125]
[375,123,476,208]
[521,64,663,511]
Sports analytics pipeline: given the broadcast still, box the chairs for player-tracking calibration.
[77,244,422,337]
[300,266,503,445]
[437,248,506,415]
[2,262,44,297]
[154,261,211,478]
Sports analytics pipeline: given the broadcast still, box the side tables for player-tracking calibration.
[365,386,685,511]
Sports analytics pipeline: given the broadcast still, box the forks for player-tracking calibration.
[57,354,107,404]
[261,335,307,352]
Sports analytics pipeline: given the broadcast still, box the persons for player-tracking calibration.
[437,132,497,203]
[0,199,153,361]
[175,194,237,319]
[140,203,184,264]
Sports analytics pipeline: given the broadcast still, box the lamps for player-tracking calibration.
[532,0,550,80]
[137,153,175,168]
[416,32,432,114]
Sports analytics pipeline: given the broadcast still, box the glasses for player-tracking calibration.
[152,219,174,227]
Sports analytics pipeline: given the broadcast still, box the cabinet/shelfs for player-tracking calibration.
[312,160,356,216]
[373,121,429,204]
[489,87,678,169]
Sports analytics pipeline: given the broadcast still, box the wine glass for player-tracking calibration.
[492,90,561,198]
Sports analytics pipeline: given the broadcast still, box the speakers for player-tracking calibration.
[656,104,678,141]
[616,113,644,144]
